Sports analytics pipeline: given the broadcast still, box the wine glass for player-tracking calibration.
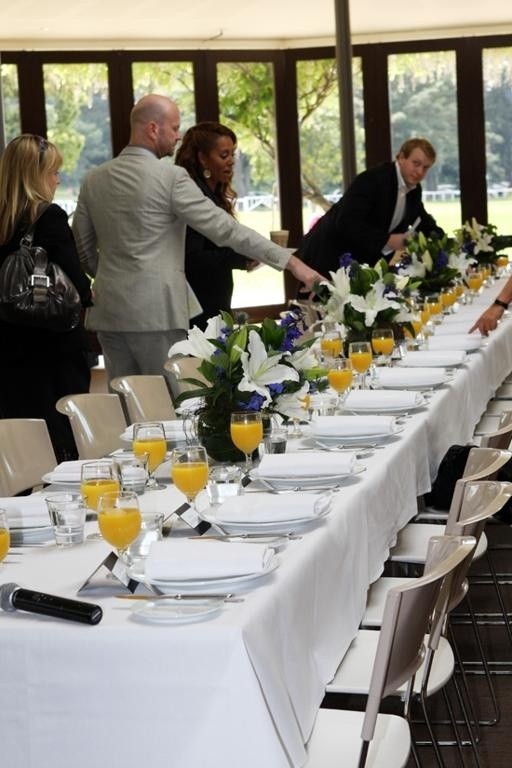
[230,408,261,483]
[314,253,512,381]
[81,416,213,569]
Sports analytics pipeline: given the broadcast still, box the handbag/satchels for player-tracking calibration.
[2,236,82,331]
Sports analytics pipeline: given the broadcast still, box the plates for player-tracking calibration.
[42,469,83,488]
[252,389,433,489]
[5,527,58,555]
[130,493,340,598]
[284,249,298,256]
[136,597,227,620]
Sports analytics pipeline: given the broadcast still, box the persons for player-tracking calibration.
[466,274,511,337]
[0,129,101,463]
[74,93,326,379]
[287,136,448,320]
[175,122,272,330]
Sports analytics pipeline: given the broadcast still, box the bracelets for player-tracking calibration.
[494,299,508,309]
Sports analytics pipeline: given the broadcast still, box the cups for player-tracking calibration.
[45,492,85,545]
[269,229,290,248]
[0,510,12,568]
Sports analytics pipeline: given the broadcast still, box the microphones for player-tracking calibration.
[0,584,102,625]
